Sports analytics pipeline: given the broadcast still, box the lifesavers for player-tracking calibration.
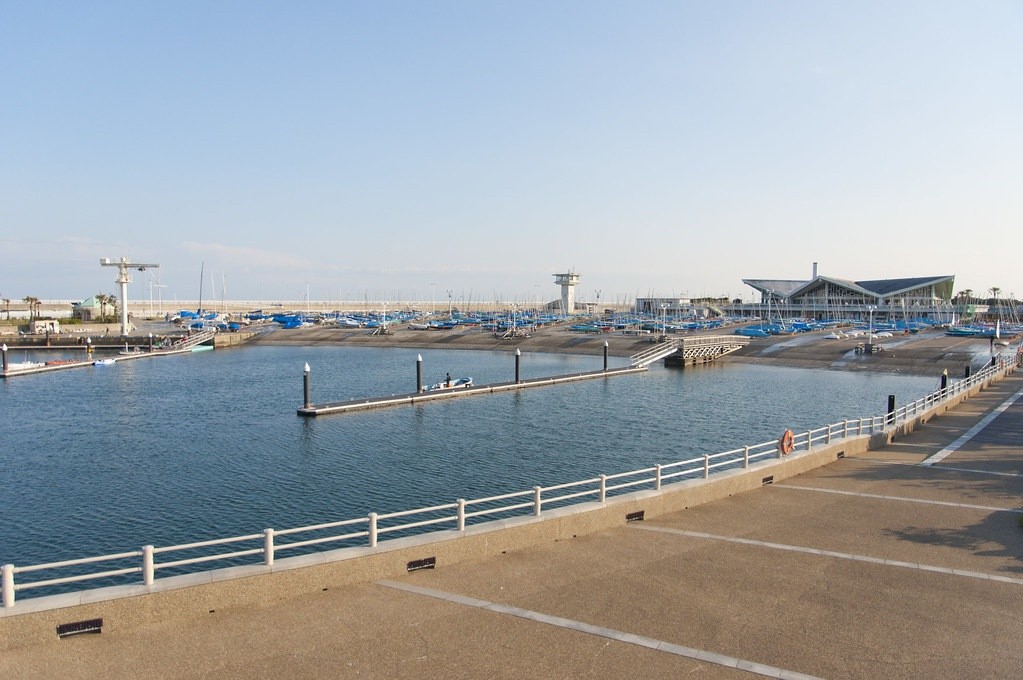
[782,429,794,455]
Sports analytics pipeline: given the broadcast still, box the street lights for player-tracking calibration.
[149,332,153,353]
[416,352,423,393]
[603,341,609,371]
[86,337,92,362]
[866,304,877,354]
[2,343,9,373]
[515,348,522,384]
[303,362,311,408]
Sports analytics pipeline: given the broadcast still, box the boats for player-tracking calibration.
[430,377,473,392]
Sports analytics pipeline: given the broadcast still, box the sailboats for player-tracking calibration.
[165,262,1023,351]
